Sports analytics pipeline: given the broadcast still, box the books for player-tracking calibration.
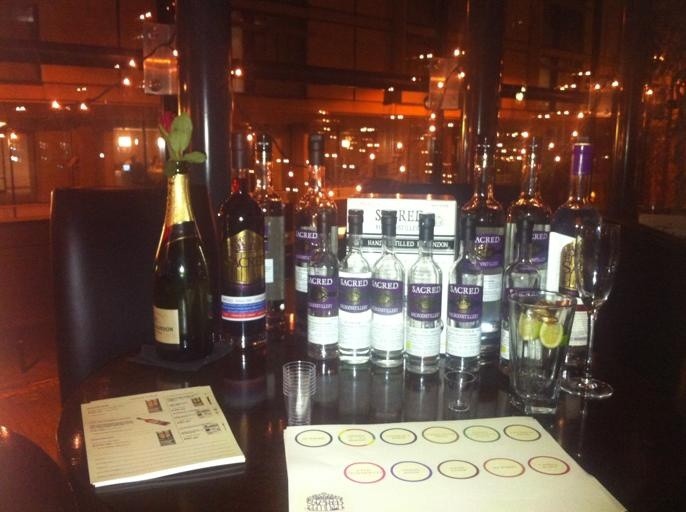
[279,412,630,512]
[80,383,246,491]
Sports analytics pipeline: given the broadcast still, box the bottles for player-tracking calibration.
[371,207,406,370]
[293,133,348,349]
[220,335,268,431]
[406,211,445,373]
[217,127,269,334]
[305,209,338,363]
[252,131,286,316]
[151,155,216,366]
[297,353,439,422]
[462,133,611,364]
[337,205,375,367]
[454,213,485,376]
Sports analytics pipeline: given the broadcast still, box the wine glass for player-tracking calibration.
[560,219,624,401]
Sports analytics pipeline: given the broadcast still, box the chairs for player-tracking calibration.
[46,184,218,406]
[2,424,74,510]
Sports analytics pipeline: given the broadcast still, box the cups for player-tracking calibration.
[443,369,477,417]
[276,360,322,428]
[506,286,579,418]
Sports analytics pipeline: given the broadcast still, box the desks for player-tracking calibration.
[54,324,686,512]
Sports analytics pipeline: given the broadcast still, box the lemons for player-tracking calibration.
[520,313,568,350]
